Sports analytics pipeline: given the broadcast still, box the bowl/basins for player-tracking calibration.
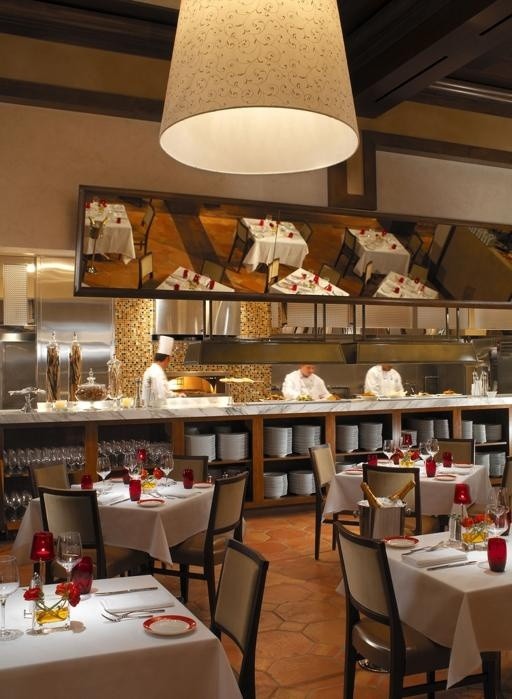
[486,391,497,397]
[34,398,132,413]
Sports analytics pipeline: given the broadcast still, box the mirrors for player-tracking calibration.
[73,183,511,310]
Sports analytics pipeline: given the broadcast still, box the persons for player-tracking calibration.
[363,363,407,397]
[143,354,187,399]
[282,363,333,403]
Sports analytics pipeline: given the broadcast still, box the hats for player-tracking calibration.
[157,335,174,356]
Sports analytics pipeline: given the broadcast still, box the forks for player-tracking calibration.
[102,607,166,624]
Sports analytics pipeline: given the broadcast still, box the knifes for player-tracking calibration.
[426,540,444,552]
[94,586,160,596]
[402,546,431,556]
[110,498,130,506]
[428,560,477,572]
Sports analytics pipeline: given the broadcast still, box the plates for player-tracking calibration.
[383,535,420,547]
[486,424,502,441]
[186,433,216,460]
[472,424,486,444]
[137,499,165,507]
[401,430,417,448]
[412,420,434,442]
[219,432,250,460]
[259,395,468,404]
[293,426,321,456]
[435,475,456,481]
[335,424,359,453]
[264,426,292,458]
[263,470,316,497]
[433,420,449,439]
[472,451,507,478]
[461,420,472,439]
[359,422,382,451]
[142,615,197,636]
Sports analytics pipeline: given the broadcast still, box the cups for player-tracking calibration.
[443,451,452,467]
[368,453,376,466]
[487,537,507,573]
[86,201,121,224]
[80,475,94,490]
[395,274,427,294]
[288,271,332,293]
[361,226,396,250]
[72,556,94,595]
[129,479,141,501]
[427,459,436,476]
[183,469,193,489]
[258,217,293,238]
[4,440,174,470]
[175,269,217,292]
[122,469,130,484]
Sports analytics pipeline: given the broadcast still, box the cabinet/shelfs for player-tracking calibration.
[0,405,511,532]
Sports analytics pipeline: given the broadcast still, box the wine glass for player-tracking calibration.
[55,532,83,631]
[6,491,33,521]
[484,483,508,538]
[159,453,174,493]
[0,555,22,643]
[95,458,112,494]
[382,433,440,474]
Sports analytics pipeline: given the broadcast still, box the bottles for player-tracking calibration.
[471,368,488,397]
[360,482,385,508]
[23,570,44,635]
[448,512,463,544]
[388,479,415,503]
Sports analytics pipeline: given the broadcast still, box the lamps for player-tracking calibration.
[156,1,362,178]
